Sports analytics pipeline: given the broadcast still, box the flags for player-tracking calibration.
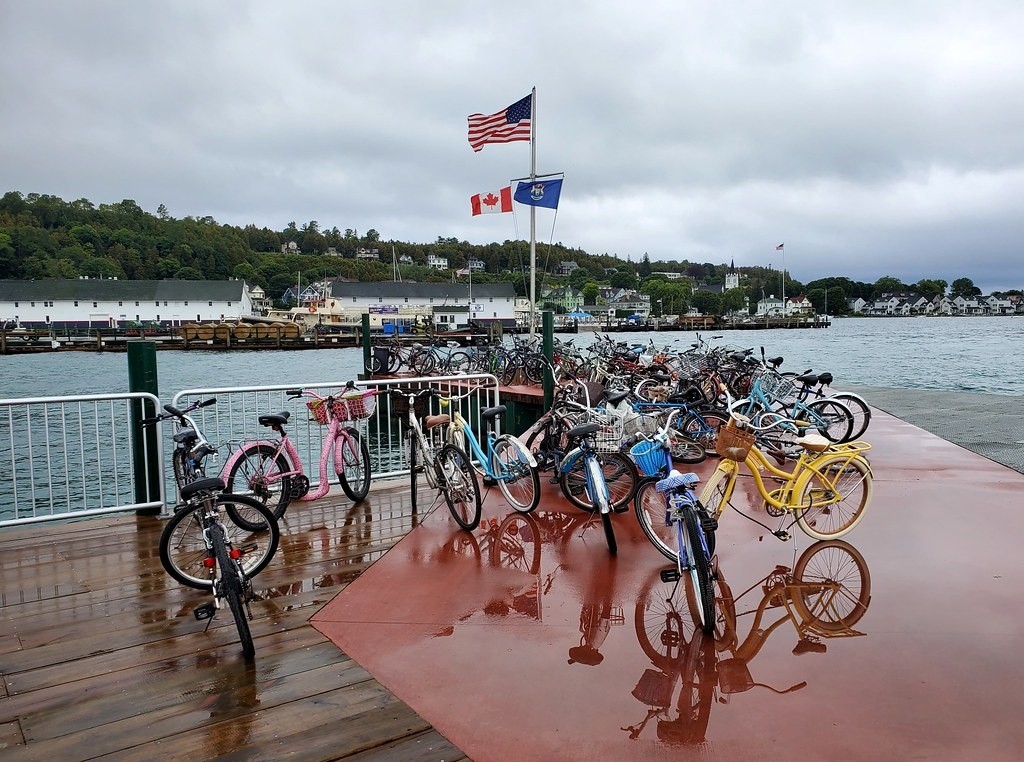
[467,93,532,153]
[776,243,784,250]
[456,265,469,277]
[470,186,513,217]
[514,179,564,210]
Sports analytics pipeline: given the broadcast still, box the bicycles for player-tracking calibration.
[365,326,871,555]
[250,499,368,616]
[623,398,721,635]
[137,396,282,660]
[220,382,379,535]
[618,535,871,745]
[372,386,483,532]
[431,503,626,669]
[427,382,543,514]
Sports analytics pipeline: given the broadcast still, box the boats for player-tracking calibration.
[266,272,354,337]
[817,313,835,321]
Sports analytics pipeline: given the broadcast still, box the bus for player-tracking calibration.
[368,313,436,335]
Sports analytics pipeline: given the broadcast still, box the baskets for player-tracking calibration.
[669,352,709,379]
[750,367,800,406]
[588,407,667,476]
[392,388,426,416]
[476,337,489,351]
[306,389,376,425]
[715,425,755,462]
